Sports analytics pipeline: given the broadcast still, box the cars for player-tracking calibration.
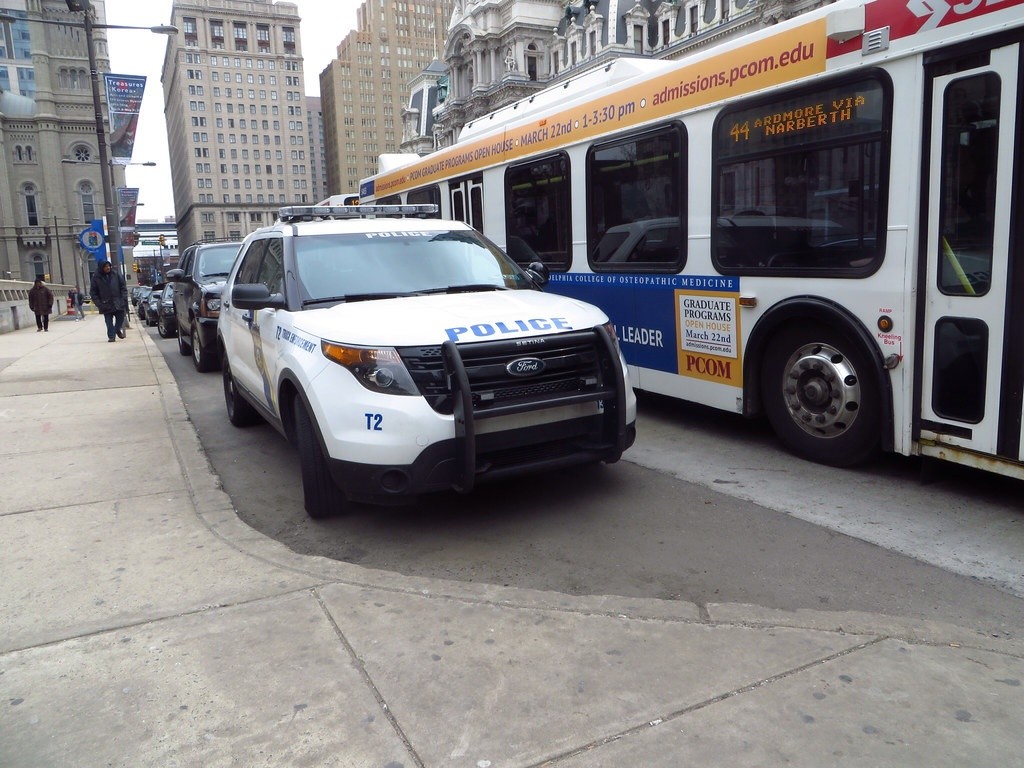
[153,281,183,338]
[141,290,165,327]
[135,287,152,320]
[130,286,143,306]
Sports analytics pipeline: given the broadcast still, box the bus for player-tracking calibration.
[315,193,358,207]
[357,0,1023,489]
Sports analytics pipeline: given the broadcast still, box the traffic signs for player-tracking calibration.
[142,242,159,245]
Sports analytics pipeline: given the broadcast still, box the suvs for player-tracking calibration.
[215,202,638,520]
[165,236,252,373]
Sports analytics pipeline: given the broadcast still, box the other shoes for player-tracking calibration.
[44,328,48,331]
[75,319,79,322]
[83,317,86,320]
[37,328,42,331]
[114,327,124,339]
[108,337,115,342]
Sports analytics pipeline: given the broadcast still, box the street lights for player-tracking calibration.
[1,12,181,328]
[60,158,156,233]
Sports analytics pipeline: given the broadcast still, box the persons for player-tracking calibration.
[504,49,516,72]
[69,287,87,321]
[91,260,129,342]
[27,278,54,332]
